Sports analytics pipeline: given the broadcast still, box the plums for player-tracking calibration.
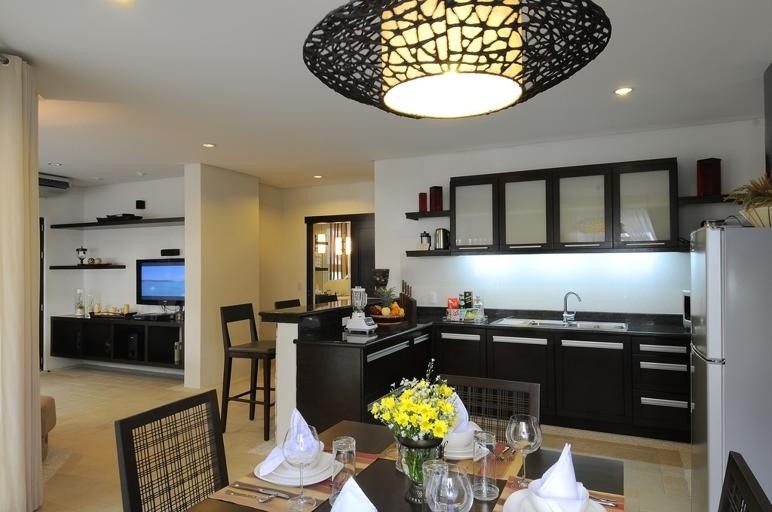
[369,306,381,315]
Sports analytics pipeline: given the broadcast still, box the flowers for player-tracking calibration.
[368,356,457,482]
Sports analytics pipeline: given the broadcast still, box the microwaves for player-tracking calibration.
[681,290,691,330]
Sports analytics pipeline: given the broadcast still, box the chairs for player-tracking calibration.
[315,294,338,305]
[275,299,301,310]
[114,389,230,512]
[220,302,276,442]
[437,374,540,434]
[715,450,771,512]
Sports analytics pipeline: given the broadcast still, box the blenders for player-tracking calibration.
[347,285,378,333]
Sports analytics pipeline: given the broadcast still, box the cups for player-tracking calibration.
[330,437,356,510]
[473,430,499,501]
[419,460,474,512]
[419,192,427,212]
[430,186,444,212]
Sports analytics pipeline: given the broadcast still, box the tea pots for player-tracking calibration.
[435,228,450,249]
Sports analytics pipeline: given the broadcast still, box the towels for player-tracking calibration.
[328,477,381,512]
[528,439,590,511]
[441,392,483,434]
[258,405,324,475]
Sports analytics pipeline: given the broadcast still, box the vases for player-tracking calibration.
[395,432,445,504]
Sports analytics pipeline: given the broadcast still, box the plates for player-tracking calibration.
[370,314,405,319]
[253,451,344,488]
[439,441,490,463]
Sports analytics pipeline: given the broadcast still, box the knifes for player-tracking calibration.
[232,479,290,499]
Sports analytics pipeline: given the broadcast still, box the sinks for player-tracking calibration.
[577,321,629,330]
[527,320,565,327]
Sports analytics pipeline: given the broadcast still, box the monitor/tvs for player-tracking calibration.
[136,258,185,306]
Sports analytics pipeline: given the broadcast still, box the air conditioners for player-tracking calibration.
[37,173,72,192]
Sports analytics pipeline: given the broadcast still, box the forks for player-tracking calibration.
[225,488,275,502]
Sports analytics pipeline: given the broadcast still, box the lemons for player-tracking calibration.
[399,308,404,315]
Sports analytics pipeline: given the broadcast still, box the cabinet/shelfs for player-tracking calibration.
[450,172,498,256]
[294,322,435,435]
[146,326,179,367]
[111,325,146,364]
[553,329,634,437]
[50,218,185,270]
[633,332,693,443]
[406,212,454,256]
[499,167,552,254]
[613,155,678,254]
[48,315,113,365]
[552,161,612,253]
[678,193,737,254]
[437,327,552,425]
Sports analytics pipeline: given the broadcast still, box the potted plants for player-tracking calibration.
[721,173,771,228]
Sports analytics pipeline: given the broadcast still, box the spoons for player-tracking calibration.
[498,447,509,461]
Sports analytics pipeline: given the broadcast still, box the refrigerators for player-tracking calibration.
[690,226,772,512]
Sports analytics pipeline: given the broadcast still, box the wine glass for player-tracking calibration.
[507,414,545,492]
[76,246,88,268]
[282,425,321,510]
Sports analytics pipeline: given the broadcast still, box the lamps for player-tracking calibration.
[303,0,612,123]
[316,231,329,256]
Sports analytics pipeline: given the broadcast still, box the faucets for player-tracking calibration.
[562,290,583,324]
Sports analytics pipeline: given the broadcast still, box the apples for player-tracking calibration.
[382,308,391,316]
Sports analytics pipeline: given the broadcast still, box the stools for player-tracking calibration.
[40,394,57,462]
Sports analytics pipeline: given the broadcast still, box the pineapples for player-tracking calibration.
[376,287,395,308]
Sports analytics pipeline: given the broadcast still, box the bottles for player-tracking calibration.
[94,303,131,316]
[420,231,432,250]
[74,287,93,318]
[696,158,723,198]
[446,291,485,321]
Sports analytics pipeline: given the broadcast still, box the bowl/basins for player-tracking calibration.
[443,420,474,449]
[283,440,324,469]
